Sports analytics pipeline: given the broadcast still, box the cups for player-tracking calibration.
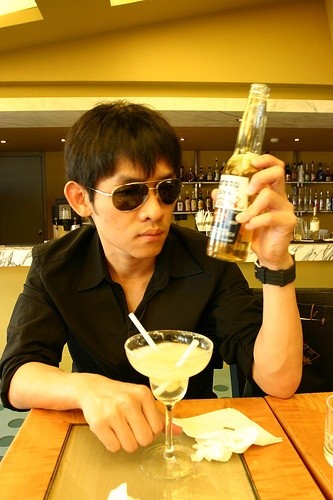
[293,217,308,240]
[323,396,333,464]
[319,229,328,240]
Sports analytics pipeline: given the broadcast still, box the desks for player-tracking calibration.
[0,393,333,500]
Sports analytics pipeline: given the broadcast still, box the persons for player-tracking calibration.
[0,101,303,454]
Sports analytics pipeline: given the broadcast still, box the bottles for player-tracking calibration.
[175,157,226,211]
[206,82,268,262]
[286,159,333,213]
[310,206,321,240]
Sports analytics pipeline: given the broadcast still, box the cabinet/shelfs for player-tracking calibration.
[173,181,333,214]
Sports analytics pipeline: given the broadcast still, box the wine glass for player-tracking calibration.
[125,329,213,479]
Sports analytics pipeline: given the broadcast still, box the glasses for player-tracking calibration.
[88,177,185,212]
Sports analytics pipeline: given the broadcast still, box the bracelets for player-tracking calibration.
[252,252,297,288]
